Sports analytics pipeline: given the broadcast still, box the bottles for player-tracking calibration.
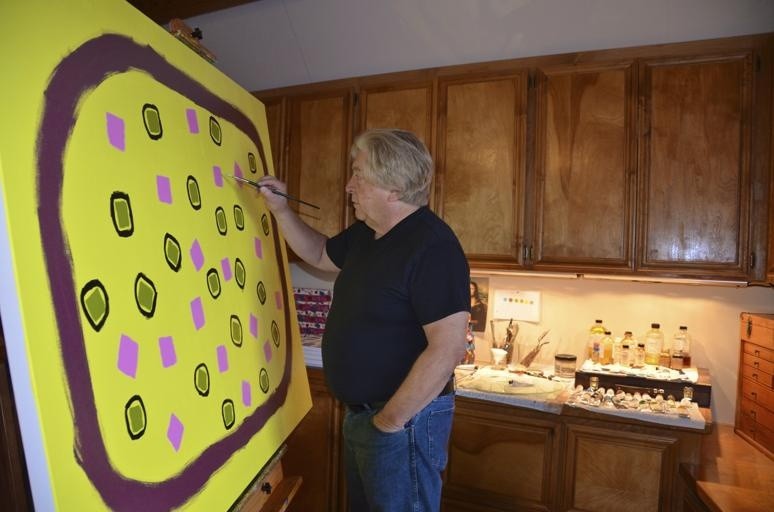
[658,348,671,368]
[669,348,684,370]
[673,325,691,356]
[619,344,631,367]
[599,331,614,365]
[587,319,606,359]
[634,342,646,367]
[643,323,665,365]
[619,330,636,364]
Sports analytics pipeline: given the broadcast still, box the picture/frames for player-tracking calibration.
[469,276,490,333]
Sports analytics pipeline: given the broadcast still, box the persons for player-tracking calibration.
[255,129,470,512]
[469,282,483,333]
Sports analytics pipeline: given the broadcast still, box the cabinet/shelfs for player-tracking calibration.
[732,311,774,461]
[356,67,527,266]
[531,52,752,276]
[257,87,349,257]
[340,374,563,512]
[556,354,712,512]
[226,442,309,512]
[284,364,333,511]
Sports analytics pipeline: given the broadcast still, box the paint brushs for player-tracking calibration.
[223,173,321,210]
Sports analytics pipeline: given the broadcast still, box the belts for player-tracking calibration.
[340,377,455,413]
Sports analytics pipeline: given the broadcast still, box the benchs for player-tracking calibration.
[681,423,774,511]
